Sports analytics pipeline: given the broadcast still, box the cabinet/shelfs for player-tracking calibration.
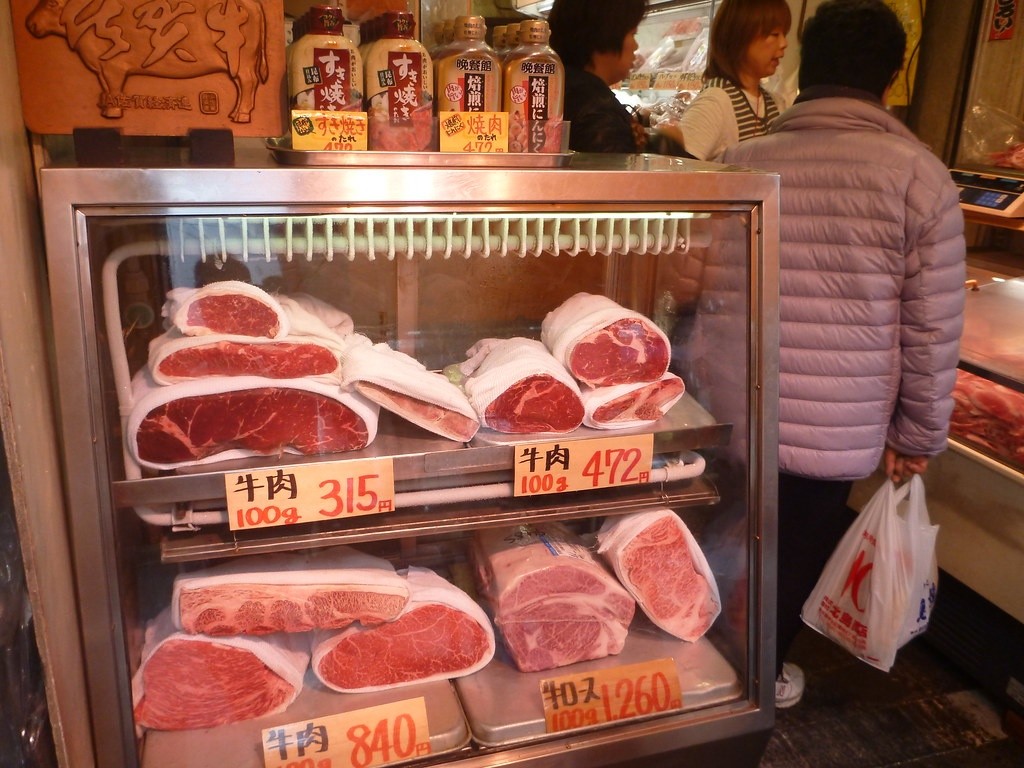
[36,154,783,768]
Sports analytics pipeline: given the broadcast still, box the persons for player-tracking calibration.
[547,0,685,160]
[677,0,794,159]
[670,1,967,709]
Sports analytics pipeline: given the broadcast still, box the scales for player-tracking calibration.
[944,163,1024,218]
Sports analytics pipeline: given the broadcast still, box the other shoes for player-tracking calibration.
[774,663,806,708]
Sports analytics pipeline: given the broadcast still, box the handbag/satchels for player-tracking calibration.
[800,473,939,674]
